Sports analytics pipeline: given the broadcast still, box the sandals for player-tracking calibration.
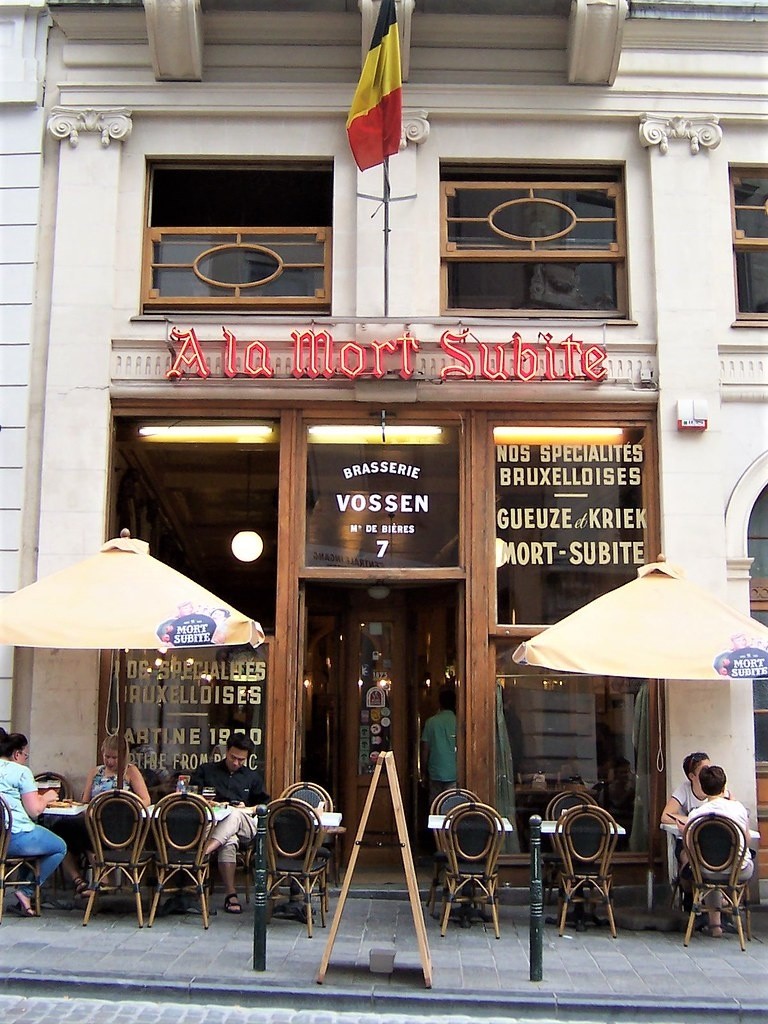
[73,877,90,893]
[701,924,722,937]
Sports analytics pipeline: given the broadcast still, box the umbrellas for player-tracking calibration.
[1,528,266,791]
[512,554,768,911]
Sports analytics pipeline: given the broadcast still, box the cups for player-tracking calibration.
[203,787,216,803]
[188,786,198,795]
[38,782,48,796]
[48,780,61,794]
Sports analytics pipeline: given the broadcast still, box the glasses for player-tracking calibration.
[689,752,701,770]
[18,749,30,760]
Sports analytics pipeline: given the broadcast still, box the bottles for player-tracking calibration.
[177,776,186,793]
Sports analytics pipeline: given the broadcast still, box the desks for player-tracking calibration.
[427,815,514,930]
[541,820,626,933]
[315,812,343,912]
[660,822,760,936]
[16,802,89,911]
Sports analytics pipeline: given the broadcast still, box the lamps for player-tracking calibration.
[134,420,275,437]
[494,426,625,440]
[230,452,265,563]
[306,424,444,437]
[495,538,509,569]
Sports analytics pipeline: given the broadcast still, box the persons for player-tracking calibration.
[0,727,67,917]
[659,753,753,938]
[419,684,457,802]
[189,733,270,915]
[589,759,636,852]
[52,735,152,899]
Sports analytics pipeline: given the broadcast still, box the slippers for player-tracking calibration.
[6,905,40,917]
[224,893,242,913]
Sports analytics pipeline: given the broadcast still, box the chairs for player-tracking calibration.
[141,803,232,917]
[438,802,505,940]
[264,797,328,939]
[546,790,599,902]
[429,788,487,921]
[324,827,346,888]
[554,804,619,939]
[34,771,75,898]
[0,793,42,923]
[82,788,151,928]
[279,783,335,812]
[209,791,272,903]
[147,791,215,930]
[682,811,752,952]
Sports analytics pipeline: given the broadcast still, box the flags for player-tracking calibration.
[346,0,404,172]
